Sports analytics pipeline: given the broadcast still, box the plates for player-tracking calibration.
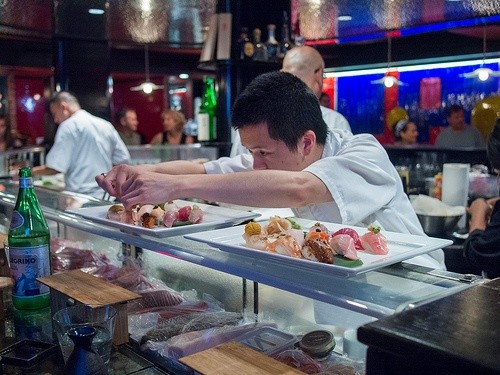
[64,199,263,237]
[184,217,454,279]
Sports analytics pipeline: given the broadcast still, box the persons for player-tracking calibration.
[435,104,482,148]
[455,119,500,281]
[32,92,130,191]
[96,72,447,271]
[0,114,27,152]
[151,110,193,144]
[114,108,141,145]
[392,119,418,144]
[228,45,350,159]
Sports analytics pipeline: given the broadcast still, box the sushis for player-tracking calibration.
[242,216,388,264]
[106,201,205,228]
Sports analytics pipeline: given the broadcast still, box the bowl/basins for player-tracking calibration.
[416,213,463,236]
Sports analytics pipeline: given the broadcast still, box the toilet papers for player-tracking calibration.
[444,164,469,227]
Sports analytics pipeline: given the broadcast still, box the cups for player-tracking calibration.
[441,163,470,232]
[51,303,119,367]
[343,330,367,375]
[394,165,410,200]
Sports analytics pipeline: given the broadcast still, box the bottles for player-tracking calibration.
[8,166,51,312]
[197,75,219,142]
[63,326,109,375]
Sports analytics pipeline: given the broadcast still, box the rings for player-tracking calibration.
[101,173,107,177]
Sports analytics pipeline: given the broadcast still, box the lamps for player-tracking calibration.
[460,23,499,81]
[371,35,405,87]
[130,46,167,94]
